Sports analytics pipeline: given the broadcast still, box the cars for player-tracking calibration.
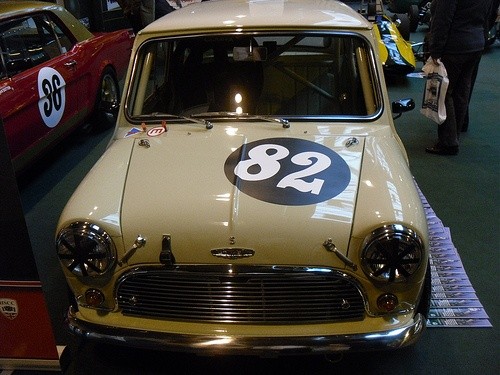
[0,0,136,171]
[58,0,431,354]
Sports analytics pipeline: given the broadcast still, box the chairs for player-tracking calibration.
[255,57,344,116]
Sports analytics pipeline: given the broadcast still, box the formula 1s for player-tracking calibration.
[324,0,432,78]
[410,0,500,53]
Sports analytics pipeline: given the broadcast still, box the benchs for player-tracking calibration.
[171,58,266,116]
[0,34,64,76]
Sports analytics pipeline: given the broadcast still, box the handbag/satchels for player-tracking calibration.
[421,56,450,125]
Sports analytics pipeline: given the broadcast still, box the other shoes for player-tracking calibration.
[426,142,459,157]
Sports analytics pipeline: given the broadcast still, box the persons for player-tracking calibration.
[423,0,500,155]
[116,0,158,39]
